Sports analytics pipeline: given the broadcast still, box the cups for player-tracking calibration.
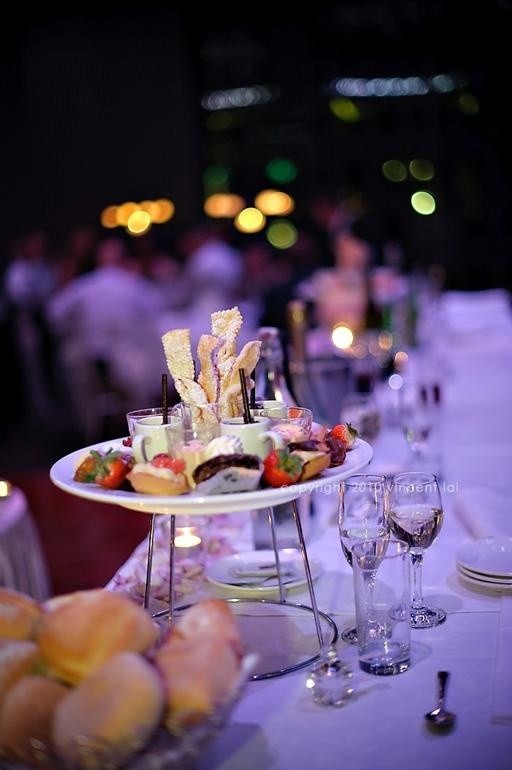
[125,400,312,467]
[158,516,212,563]
[352,539,413,677]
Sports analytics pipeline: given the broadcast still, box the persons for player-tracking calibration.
[5,205,418,411]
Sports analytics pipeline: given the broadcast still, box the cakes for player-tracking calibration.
[191,434,266,495]
[125,453,188,496]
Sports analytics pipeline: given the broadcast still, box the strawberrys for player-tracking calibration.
[73,447,132,489]
[263,445,303,487]
[332,422,357,449]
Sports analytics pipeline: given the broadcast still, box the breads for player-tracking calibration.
[0,585,245,770]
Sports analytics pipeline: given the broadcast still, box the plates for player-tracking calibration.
[456,535,512,592]
[45,419,376,517]
[207,547,324,594]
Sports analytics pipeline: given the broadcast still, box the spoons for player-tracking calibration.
[424,670,456,730]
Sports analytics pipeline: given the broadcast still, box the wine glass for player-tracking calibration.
[340,473,447,646]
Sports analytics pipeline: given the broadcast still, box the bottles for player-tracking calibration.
[308,643,355,710]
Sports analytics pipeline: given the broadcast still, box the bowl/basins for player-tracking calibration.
[3,648,264,769]
